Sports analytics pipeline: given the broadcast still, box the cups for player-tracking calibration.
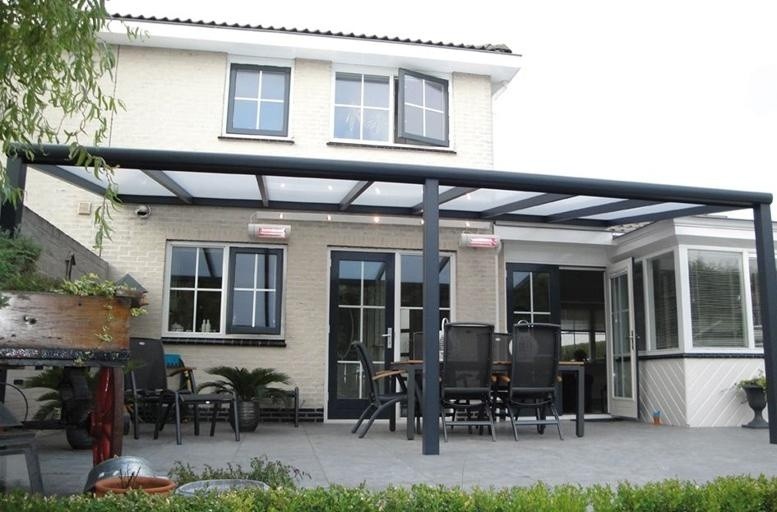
[653,412,662,425]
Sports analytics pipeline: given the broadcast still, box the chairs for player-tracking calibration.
[412,330,424,437]
[350,339,415,434]
[488,333,519,422]
[1,383,49,495]
[511,323,565,440]
[438,323,500,444]
[129,339,242,446]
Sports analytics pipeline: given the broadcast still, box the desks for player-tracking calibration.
[389,358,586,437]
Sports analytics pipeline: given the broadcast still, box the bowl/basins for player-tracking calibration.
[83,456,157,494]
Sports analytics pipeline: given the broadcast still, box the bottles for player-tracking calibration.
[201,318,212,332]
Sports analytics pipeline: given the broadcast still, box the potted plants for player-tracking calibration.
[729,369,772,430]
[201,363,291,433]
